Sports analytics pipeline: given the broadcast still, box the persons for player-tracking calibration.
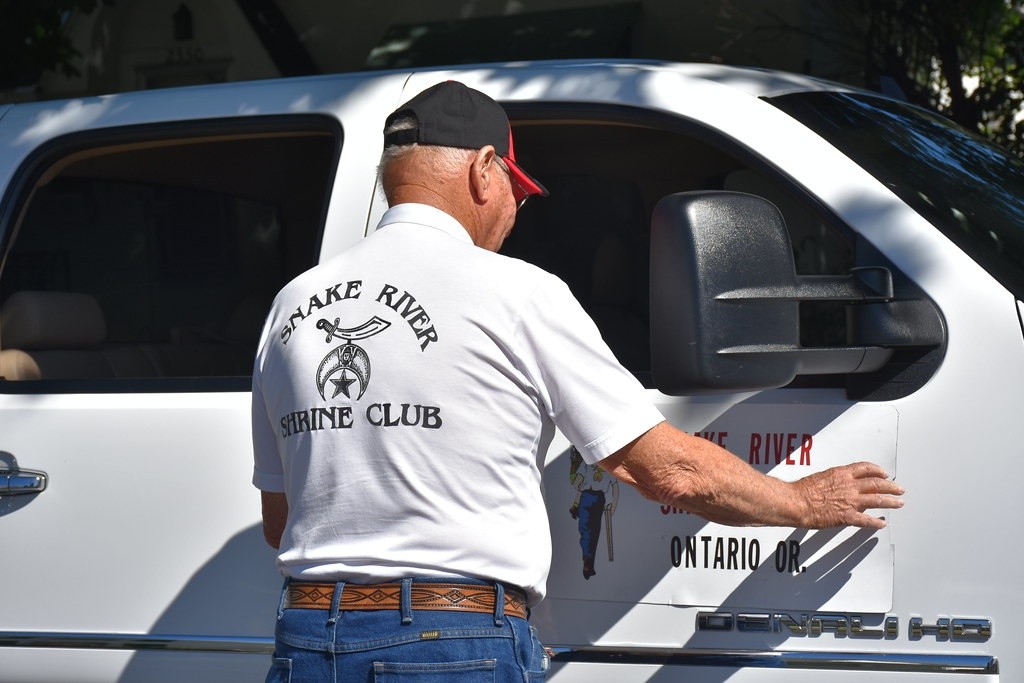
[252,79,905,683]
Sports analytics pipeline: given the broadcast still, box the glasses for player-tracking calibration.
[470,157,529,211]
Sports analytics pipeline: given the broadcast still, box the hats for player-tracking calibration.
[383,80,549,204]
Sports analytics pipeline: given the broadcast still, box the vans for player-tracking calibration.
[0,60,1024,683]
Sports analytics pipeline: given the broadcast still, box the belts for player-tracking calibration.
[282,582,528,623]
